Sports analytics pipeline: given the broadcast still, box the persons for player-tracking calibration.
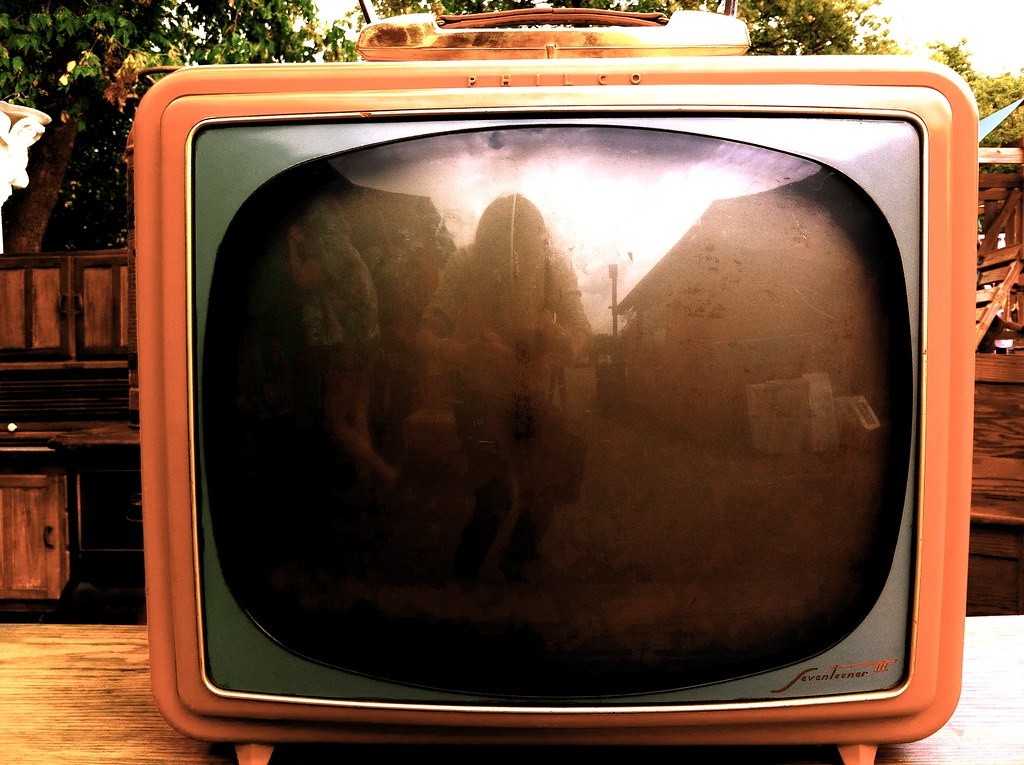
[288,153,600,588]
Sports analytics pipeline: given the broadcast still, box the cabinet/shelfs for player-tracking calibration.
[0,248,130,370]
[0,467,78,611]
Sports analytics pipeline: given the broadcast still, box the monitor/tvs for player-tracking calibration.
[131,51,979,765]
